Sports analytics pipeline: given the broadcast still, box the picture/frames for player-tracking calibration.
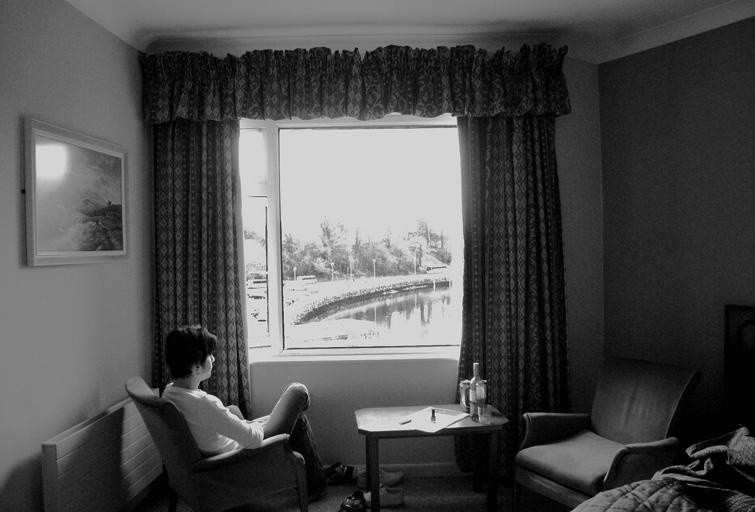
[23,117,132,269]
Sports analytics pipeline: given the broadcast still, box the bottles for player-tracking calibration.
[469,362,486,422]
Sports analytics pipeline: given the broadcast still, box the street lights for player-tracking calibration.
[331,262,335,281]
[292,267,297,280]
[373,259,377,278]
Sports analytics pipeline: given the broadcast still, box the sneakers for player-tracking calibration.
[323,462,357,483]
[363,484,404,508]
[357,467,403,488]
[338,490,366,511]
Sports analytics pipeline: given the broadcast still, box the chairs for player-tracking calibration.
[513,357,705,512]
[124,376,309,512]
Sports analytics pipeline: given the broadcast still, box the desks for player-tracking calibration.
[355,405,511,512]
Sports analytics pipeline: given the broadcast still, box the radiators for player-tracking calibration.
[41,384,164,512]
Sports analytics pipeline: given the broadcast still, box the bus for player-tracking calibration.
[247,275,318,291]
[427,265,448,274]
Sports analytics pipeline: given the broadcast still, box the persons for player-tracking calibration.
[160,322,330,504]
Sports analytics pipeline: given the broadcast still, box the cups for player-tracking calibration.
[478,405,492,424]
